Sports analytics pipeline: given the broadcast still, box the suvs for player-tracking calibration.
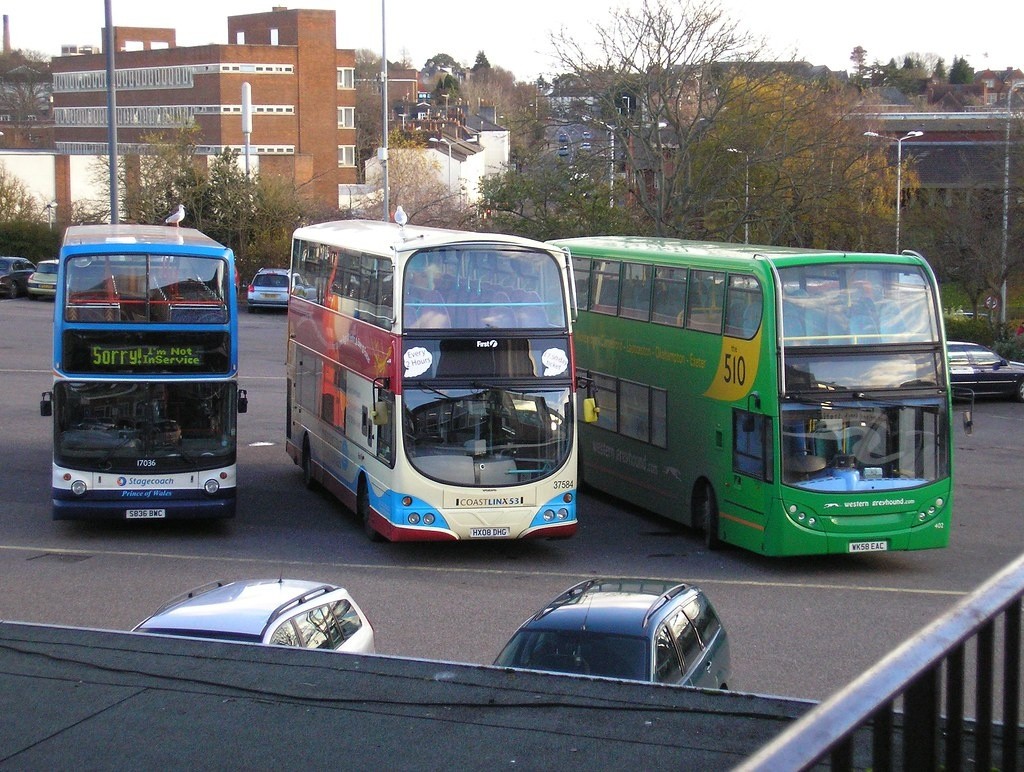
[559,134,568,142]
[495,579,730,693]
[131,579,376,658]
[246,267,292,313]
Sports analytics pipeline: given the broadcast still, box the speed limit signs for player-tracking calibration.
[985,295,999,310]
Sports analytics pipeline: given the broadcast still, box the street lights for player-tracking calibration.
[241,81,253,178]
[863,130,923,255]
[726,147,756,245]
[429,136,478,196]
[582,115,668,210]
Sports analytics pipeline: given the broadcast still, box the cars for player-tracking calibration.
[0,256,37,299]
[559,146,569,155]
[26,259,60,301]
[580,143,591,152]
[946,340,1023,403]
[583,132,590,140]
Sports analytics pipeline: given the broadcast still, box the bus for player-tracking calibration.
[40,224,248,522]
[285,219,600,542]
[542,237,975,556]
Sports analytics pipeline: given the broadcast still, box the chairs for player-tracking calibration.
[840,427,867,455]
[543,654,590,674]
[575,274,908,345]
[760,425,802,482]
[812,428,839,460]
[350,268,549,328]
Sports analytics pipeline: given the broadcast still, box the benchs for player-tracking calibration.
[66,264,227,323]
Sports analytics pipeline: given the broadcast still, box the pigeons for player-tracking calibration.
[394,206,407,227]
[165,204,185,227]
